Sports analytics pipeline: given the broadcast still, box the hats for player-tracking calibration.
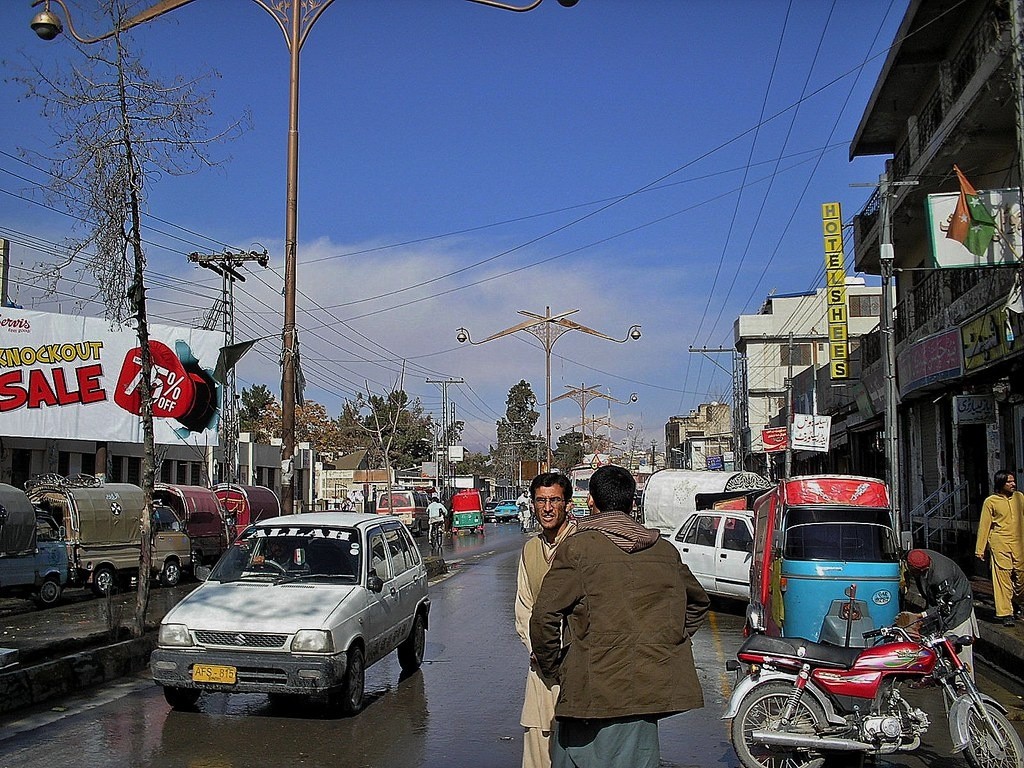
[907,550,930,570]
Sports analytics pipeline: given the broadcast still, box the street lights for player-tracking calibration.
[31,0,578,526]
[455,306,644,481]
[528,383,639,462]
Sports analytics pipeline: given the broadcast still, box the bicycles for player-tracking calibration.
[429,521,444,547]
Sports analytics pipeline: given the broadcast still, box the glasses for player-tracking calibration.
[531,498,569,507]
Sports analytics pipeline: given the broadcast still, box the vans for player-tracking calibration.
[376,486,428,538]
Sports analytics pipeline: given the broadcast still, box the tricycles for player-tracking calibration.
[451,489,484,535]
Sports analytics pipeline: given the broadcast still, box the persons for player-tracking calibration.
[516,491,541,533]
[975,470,1024,626]
[252,538,312,576]
[514,472,579,768]
[426,497,448,544]
[904,548,980,690]
[529,465,710,768]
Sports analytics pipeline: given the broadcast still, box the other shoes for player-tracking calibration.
[429,541,432,544]
[1003,617,1015,627]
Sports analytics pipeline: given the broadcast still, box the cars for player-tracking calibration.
[484,501,499,519]
[494,500,519,523]
[150,511,432,716]
[668,509,755,601]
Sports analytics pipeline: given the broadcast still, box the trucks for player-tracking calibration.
[22,473,193,597]
[150,482,237,579]
[209,484,284,543]
[0,483,72,607]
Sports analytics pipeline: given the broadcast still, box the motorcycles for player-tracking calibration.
[722,580,1024,767]
[749,473,917,651]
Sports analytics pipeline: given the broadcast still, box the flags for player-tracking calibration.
[946,165,996,256]
